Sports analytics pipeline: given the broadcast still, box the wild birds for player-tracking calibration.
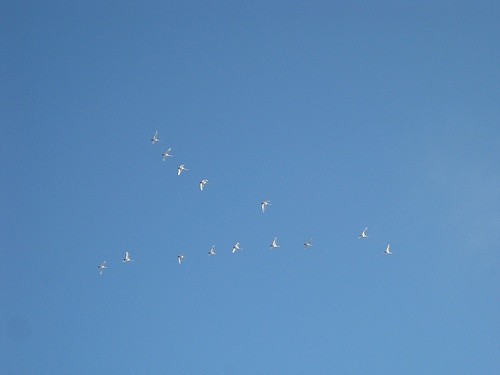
[98,130,394,275]
